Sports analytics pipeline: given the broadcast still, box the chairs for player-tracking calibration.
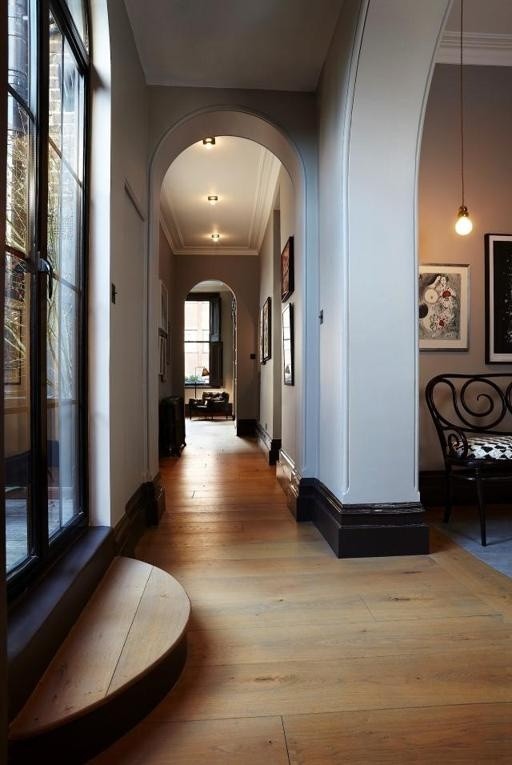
[425,372,512,546]
[189,392,232,420]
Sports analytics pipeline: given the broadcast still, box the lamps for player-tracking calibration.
[455,1,472,236]
[195,366,210,398]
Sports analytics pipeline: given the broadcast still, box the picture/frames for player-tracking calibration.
[158,280,171,383]
[259,236,294,388]
[420,233,512,365]
[3,305,21,386]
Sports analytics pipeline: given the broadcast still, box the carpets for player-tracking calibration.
[428,517,511,581]
